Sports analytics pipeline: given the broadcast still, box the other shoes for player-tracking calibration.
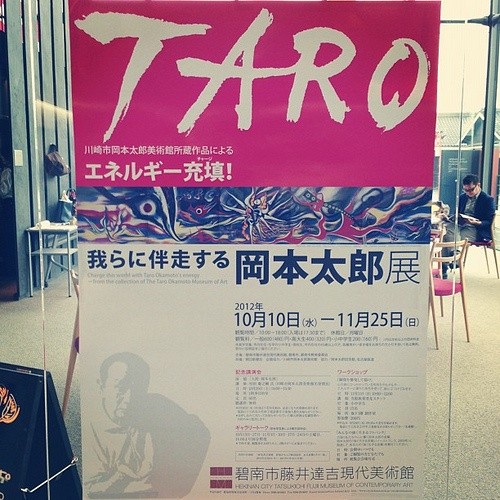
[449,262,460,268]
[435,275,447,279]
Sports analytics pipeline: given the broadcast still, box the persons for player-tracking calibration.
[80,349,157,498]
[437,173,495,279]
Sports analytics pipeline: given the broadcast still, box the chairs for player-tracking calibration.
[462,211,500,279]
[430,229,444,279]
[428,238,470,350]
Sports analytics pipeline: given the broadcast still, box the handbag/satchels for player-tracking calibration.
[55,188,75,222]
[46,146,71,176]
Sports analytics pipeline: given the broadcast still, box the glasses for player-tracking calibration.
[462,185,476,192]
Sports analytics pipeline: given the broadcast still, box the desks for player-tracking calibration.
[26,225,78,297]
